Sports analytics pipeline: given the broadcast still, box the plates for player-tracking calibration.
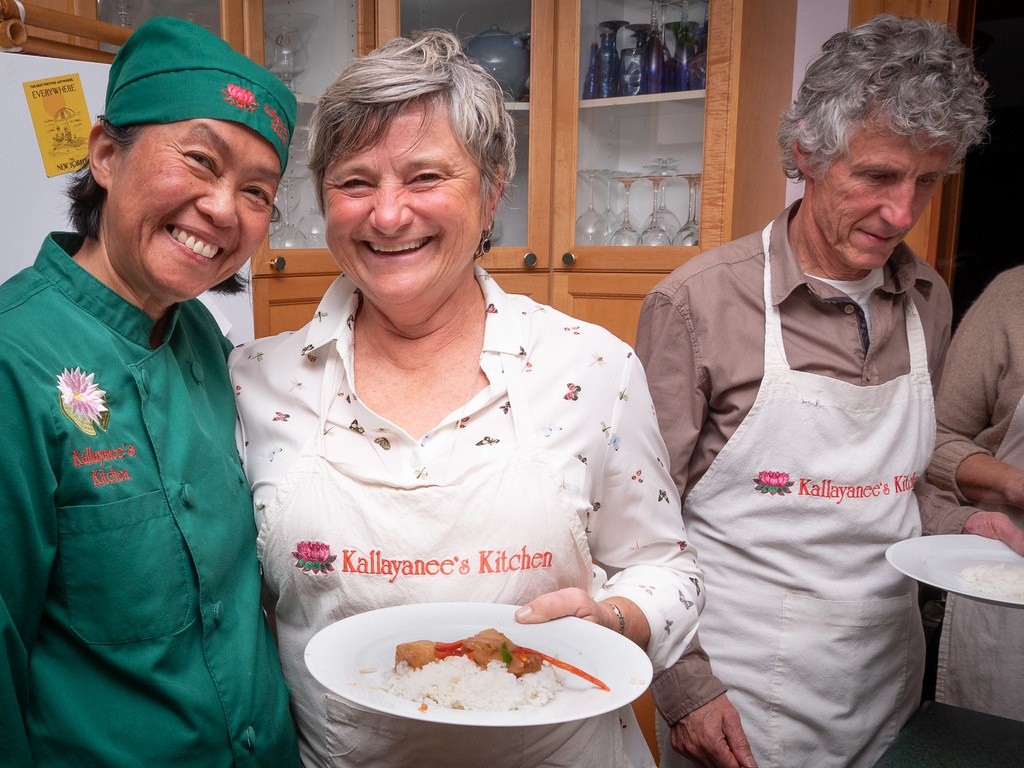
[885,533,1024,610]
[304,602,654,727]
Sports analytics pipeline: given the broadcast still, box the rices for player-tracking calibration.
[955,562,1024,598]
[360,655,567,711]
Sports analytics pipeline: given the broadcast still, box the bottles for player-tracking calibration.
[583,29,699,103]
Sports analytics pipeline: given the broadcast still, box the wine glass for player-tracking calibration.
[600,0,710,98]
[266,157,328,250]
[262,11,319,93]
[110,0,134,27]
[574,169,702,247]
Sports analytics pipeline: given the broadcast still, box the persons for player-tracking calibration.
[633,13,1024,768]
[925,264,1024,768]
[0,16,297,768]
[228,28,705,768]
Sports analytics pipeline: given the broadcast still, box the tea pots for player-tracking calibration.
[458,25,531,102]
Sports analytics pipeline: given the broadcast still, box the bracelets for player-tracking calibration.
[603,599,625,639]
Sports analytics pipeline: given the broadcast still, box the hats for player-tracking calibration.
[104,15,297,176]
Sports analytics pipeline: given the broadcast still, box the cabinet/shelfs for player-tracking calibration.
[0,1,798,350]
